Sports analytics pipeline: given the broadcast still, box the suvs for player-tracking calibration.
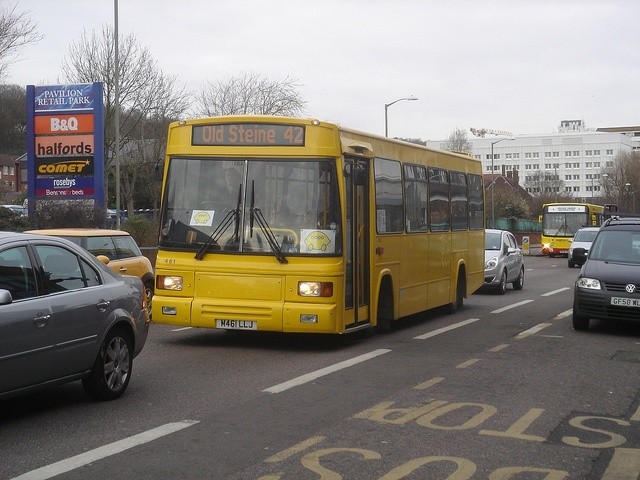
[573,215,640,330]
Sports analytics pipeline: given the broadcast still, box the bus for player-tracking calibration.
[151,115,485,335]
[539,203,603,257]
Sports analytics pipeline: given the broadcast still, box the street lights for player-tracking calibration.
[491,137,515,227]
[385,98,418,137]
[591,174,608,203]
[616,183,630,214]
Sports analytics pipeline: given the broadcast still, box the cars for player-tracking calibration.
[568,225,600,267]
[0,232,147,400]
[485,229,524,294]
[0,205,23,217]
[24,228,154,320]
